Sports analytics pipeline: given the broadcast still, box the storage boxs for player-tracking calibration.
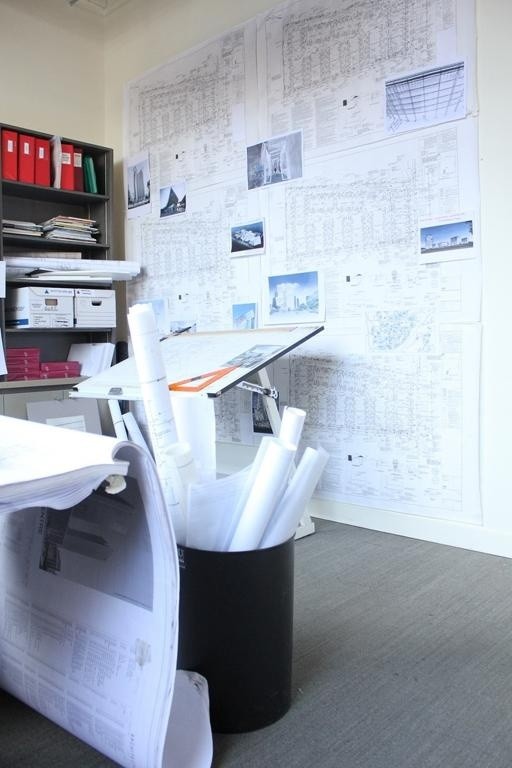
[6,285,118,331]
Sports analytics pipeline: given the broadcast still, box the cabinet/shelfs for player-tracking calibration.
[0,120,115,441]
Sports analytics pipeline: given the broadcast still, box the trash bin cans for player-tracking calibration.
[177,534,294,734]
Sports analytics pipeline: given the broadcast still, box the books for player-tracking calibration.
[0,414,226,767]
[2,214,102,246]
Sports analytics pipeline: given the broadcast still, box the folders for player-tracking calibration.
[2,129,85,192]
